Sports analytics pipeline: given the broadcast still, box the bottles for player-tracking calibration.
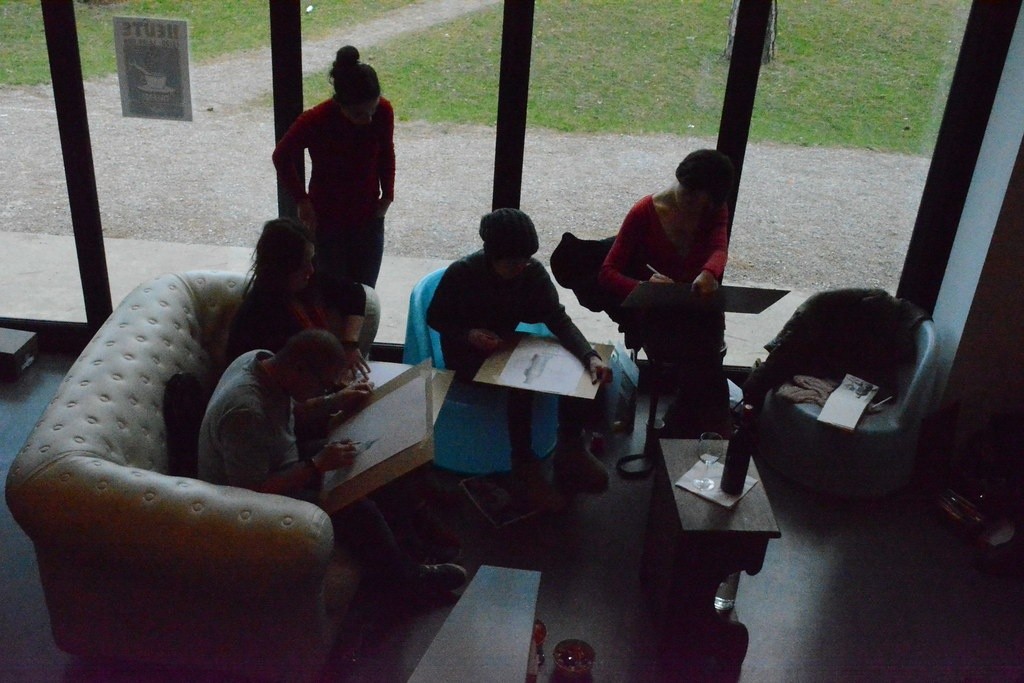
[721,397,761,496]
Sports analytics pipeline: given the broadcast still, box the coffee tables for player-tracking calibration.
[640,438,780,662]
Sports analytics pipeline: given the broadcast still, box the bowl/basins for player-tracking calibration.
[553,639,595,679]
[532,618,547,647]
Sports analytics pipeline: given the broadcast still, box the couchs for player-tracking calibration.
[403,270,559,473]
[5,271,379,683]
[766,288,941,500]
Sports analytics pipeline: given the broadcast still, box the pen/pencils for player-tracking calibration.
[647,263,661,276]
[324,441,361,447]
[480,332,504,342]
[872,395,894,408]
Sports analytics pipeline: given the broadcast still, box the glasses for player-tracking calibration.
[307,360,334,393]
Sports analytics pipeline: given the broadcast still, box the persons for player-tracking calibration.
[427,208,611,498]
[270,44,395,292]
[194,217,467,600]
[599,149,737,453]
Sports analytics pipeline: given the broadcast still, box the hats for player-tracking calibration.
[481,207,538,258]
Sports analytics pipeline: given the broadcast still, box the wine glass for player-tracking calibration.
[694,432,723,490]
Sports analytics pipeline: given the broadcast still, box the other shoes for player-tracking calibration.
[416,564,466,592]
[698,417,733,437]
[463,474,512,511]
[555,444,608,483]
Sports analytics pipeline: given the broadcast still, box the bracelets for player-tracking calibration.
[311,457,327,477]
[341,339,359,350]
